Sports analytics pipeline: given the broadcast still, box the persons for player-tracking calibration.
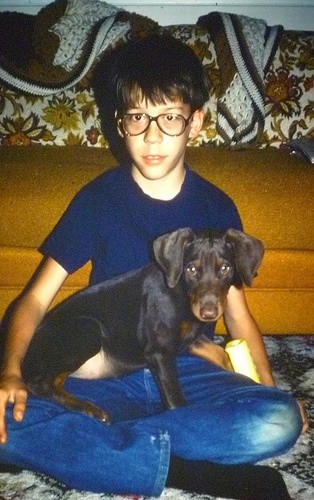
[0,34,308,500]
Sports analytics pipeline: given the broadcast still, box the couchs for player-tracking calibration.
[0,0,314,334]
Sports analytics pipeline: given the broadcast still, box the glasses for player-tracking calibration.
[121,111,195,137]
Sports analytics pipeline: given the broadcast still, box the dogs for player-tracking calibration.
[22,227,265,423]
